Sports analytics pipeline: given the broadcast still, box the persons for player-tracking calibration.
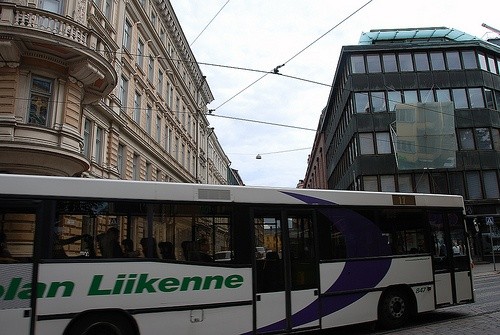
[107,227,140,257]
[435,240,446,257]
[453,241,466,255]
[50,212,86,257]
[198,238,214,263]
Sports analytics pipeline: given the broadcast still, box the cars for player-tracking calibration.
[255,247,266,259]
[210,251,234,263]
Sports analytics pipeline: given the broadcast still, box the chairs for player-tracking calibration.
[97,233,106,257]
[84,234,95,258]
[122,238,134,256]
[141,237,157,257]
[159,242,175,259]
[181,241,196,262]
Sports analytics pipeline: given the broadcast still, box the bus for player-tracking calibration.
[0,173,475,335]
